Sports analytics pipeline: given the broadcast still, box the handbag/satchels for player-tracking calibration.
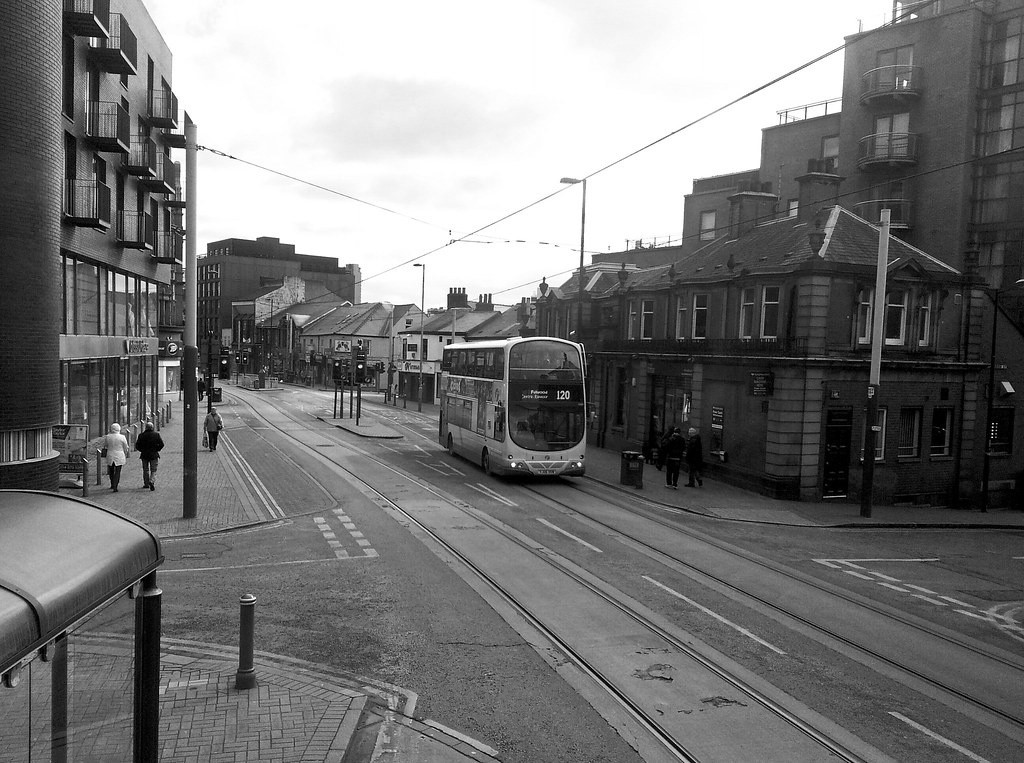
[217,423,222,430]
[202,432,209,447]
[101,445,108,458]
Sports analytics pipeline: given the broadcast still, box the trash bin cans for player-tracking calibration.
[210,387,222,403]
[620,451,645,486]
[255,379,259,389]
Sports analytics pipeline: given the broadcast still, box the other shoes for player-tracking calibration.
[213,446,216,450]
[672,485,679,489]
[210,448,213,452]
[685,483,695,487]
[149,482,155,491]
[111,485,118,492]
[143,485,149,487]
[700,482,703,487]
[665,485,671,488]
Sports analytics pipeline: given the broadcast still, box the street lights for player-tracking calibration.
[981,279,1024,514]
[560,178,586,344]
[265,299,273,377]
[414,264,425,413]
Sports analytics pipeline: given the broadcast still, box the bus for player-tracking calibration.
[438,336,598,479]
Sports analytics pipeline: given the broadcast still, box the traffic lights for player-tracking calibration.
[235,352,241,364]
[332,360,342,380]
[242,352,248,365]
[356,360,366,384]
[218,356,231,380]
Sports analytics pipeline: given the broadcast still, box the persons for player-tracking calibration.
[204,407,224,452]
[105,422,129,492]
[135,422,165,491]
[645,423,662,466]
[684,428,703,488]
[663,427,686,490]
[197,377,205,402]
